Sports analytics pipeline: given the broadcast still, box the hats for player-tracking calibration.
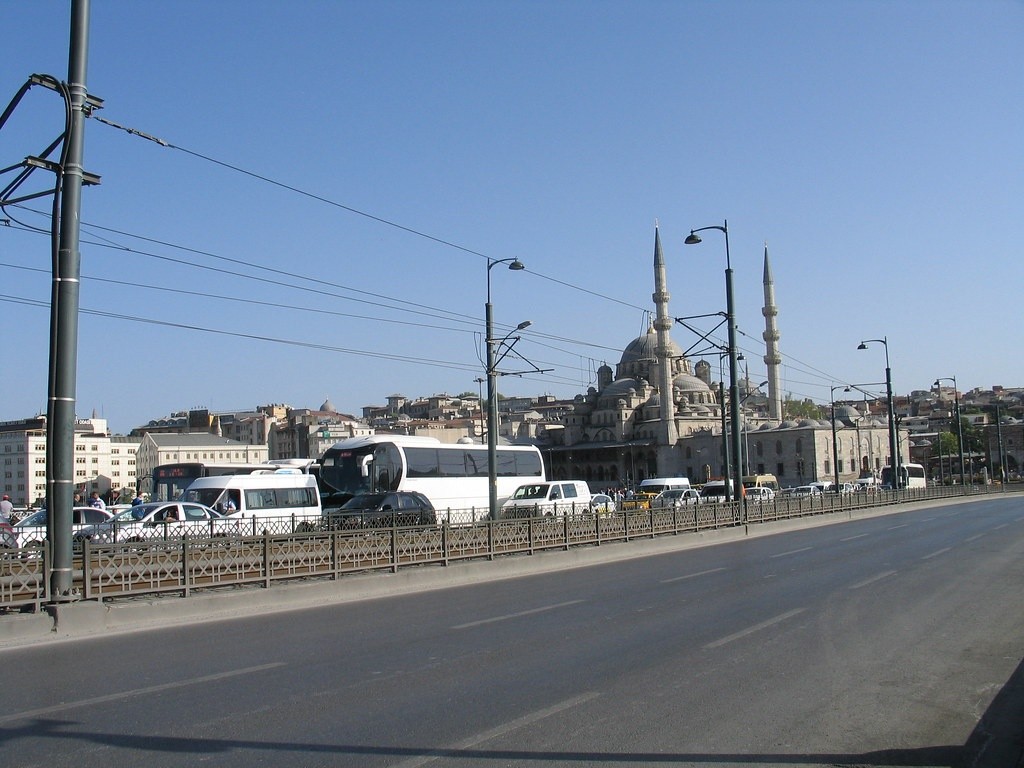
[3,495,8,500]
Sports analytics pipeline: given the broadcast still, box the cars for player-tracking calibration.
[650,489,702,511]
[746,487,775,503]
[0,515,18,561]
[590,493,616,519]
[774,478,894,501]
[11,506,114,562]
[622,493,659,515]
[72,502,240,555]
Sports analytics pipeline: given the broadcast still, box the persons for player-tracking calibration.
[163,508,177,522]
[223,495,236,513]
[598,487,638,503]
[73,492,106,510]
[0,495,13,518]
[131,491,146,520]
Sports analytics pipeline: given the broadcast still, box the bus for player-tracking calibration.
[305,434,547,530]
[879,463,926,490]
[136,459,322,503]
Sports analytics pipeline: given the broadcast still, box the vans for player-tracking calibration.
[501,480,591,523]
[742,474,778,492]
[640,477,691,497]
[163,468,323,543]
[700,479,734,503]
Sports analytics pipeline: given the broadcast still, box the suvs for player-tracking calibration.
[322,490,438,539]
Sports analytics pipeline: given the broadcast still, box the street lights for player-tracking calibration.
[856,336,898,490]
[720,351,769,503]
[933,375,966,486]
[830,385,851,496]
[483,255,532,525]
[683,219,743,505]
